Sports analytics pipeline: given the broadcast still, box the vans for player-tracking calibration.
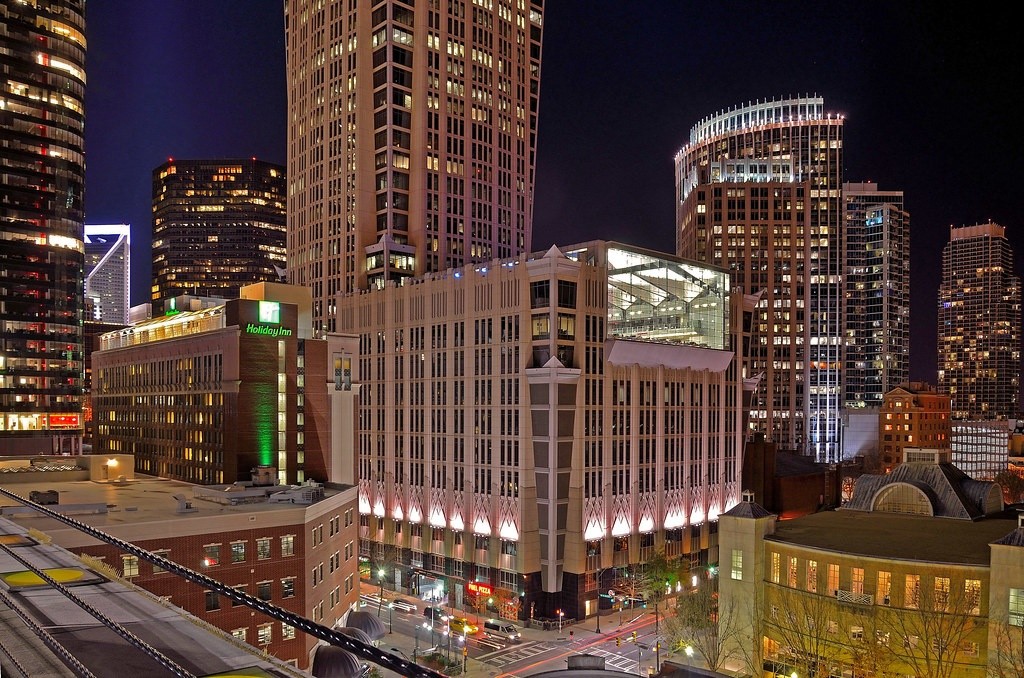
[483,618,522,645]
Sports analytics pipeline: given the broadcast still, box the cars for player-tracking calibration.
[423,605,455,624]
[391,598,418,616]
[447,617,479,636]
